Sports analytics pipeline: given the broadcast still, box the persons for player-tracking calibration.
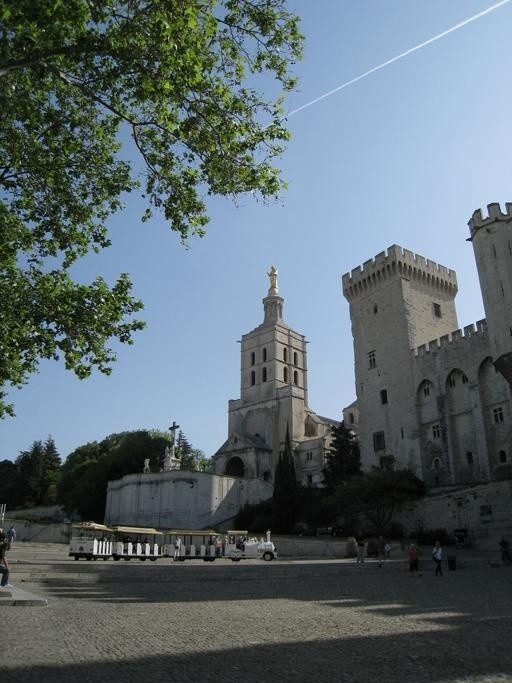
[0,527,6,543]
[7,526,16,542]
[174,536,181,561]
[0,543,13,588]
[432,541,443,577]
[498,535,511,565]
[407,544,422,577]
[80,533,148,553]
[357,537,365,563]
[376,536,387,561]
[208,534,264,556]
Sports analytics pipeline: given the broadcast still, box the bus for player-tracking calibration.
[69,521,277,561]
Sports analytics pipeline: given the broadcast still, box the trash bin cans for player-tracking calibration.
[447,555,456,570]
